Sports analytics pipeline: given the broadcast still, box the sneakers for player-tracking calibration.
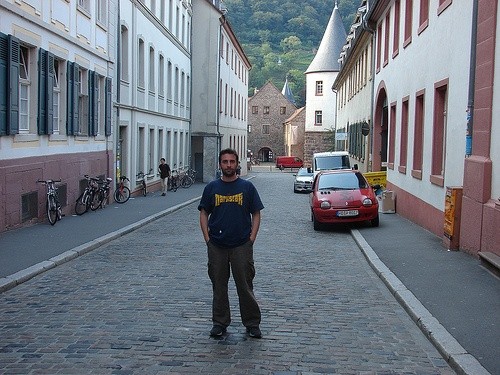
[210,325,226,336]
[246,326,261,337]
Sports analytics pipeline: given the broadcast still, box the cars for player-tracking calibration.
[308,168,380,232]
[292,167,314,193]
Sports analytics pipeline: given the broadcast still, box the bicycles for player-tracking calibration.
[166,162,196,192]
[136,172,151,197]
[74,174,112,216]
[113,175,130,204]
[36,177,63,225]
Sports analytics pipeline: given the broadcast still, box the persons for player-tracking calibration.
[198,148,265,337]
[157,158,170,196]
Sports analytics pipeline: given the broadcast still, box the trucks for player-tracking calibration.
[311,150,358,183]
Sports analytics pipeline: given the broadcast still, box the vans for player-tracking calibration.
[275,156,304,170]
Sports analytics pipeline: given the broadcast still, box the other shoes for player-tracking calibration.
[161,193,166,196]
